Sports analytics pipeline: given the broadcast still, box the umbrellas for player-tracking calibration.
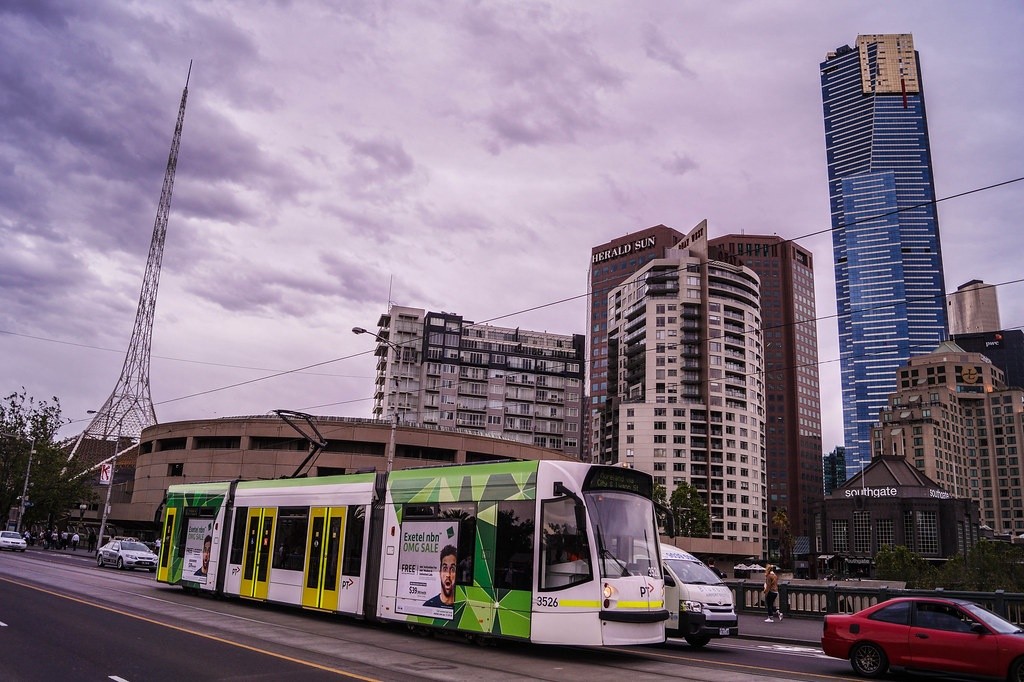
[734,564,766,572]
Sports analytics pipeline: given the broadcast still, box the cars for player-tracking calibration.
[0,531,27,552]
[821,597,1024,682]
[97,536,157,572]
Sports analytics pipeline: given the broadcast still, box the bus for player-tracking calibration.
[155,409,666,649]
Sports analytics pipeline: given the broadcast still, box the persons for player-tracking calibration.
[763,564,783,623]
[87,531,97,553]
[423,545,457,609]
[193,535,212,577]
[21,529,37,546]
[155,534,163,556]
[708,556,723,580]
[71,533,80,551]
[502,562,514,590]
[561,535,585,560]
[39,527,73,550]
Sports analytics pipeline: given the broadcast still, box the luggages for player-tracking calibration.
[43,541,48,549]
[55,540,61,550]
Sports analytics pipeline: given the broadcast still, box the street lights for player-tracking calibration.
[87,410,123,556]
[0,432,36,532]
[352,327,406,470]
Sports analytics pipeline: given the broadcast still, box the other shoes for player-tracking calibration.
[764,616,774,622]
[778,613,783,621]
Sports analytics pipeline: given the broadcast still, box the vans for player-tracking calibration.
[611,539,738,647]
